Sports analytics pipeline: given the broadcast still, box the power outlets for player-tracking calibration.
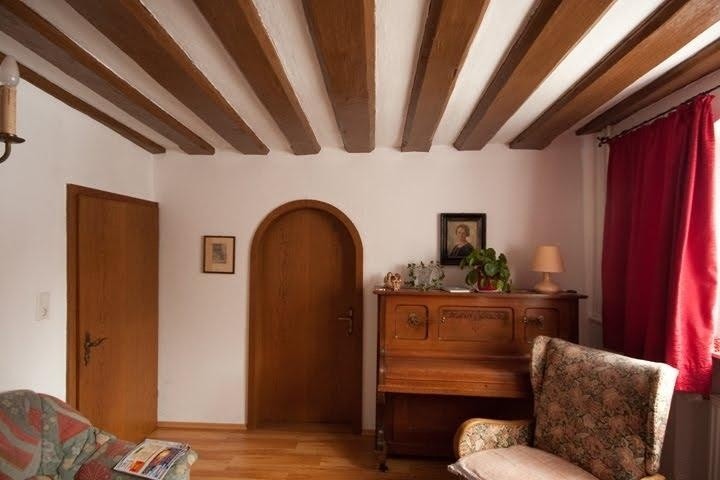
[33,292,50,321]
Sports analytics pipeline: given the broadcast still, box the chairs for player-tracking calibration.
[455,335,679,480]
[1,390,199,480]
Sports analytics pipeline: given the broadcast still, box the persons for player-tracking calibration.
[449,224,474,259]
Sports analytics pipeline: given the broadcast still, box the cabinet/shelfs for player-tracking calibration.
[374,286,590,473]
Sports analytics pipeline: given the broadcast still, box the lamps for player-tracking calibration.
[0,56,25,163]
[532,244,564,292]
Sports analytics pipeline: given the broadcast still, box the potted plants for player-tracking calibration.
[405,261,446,293]
[460,248,513,294]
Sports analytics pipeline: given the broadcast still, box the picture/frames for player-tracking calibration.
[440,213,487,265]
[203,236,235,274]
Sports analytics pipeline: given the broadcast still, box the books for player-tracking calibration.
[113,438,191,480]
[439,285,471,295]
